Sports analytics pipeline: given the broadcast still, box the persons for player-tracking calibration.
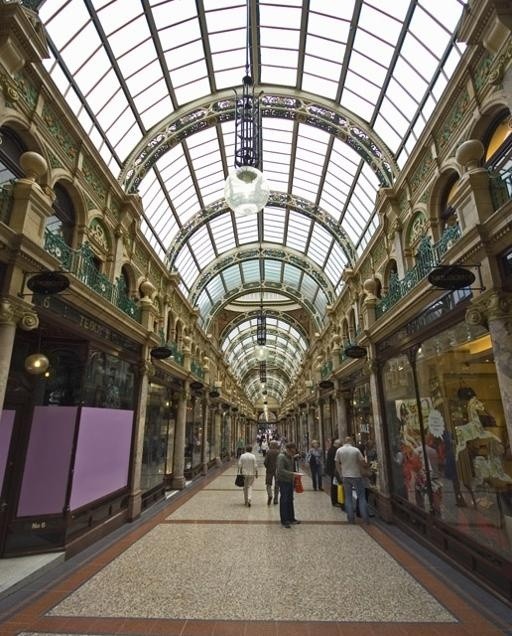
[275,441,304,528]
[393,436,441,513]
[237,429,376,518]
[334,436,369,522]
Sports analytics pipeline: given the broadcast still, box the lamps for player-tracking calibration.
[222,1,270,219]
[260,359,266,391]
[255,297,268,363]
[24,331,50,375]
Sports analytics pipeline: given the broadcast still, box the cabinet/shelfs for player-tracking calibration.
[434,362,512,486]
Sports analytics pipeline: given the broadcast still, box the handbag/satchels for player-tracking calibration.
[295,475,304,492]
[235,475,244,486]
[337,484,344,504]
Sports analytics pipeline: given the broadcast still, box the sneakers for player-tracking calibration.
[282,520,291,528]
[289,519,301,524]
[248,497,278,507]
